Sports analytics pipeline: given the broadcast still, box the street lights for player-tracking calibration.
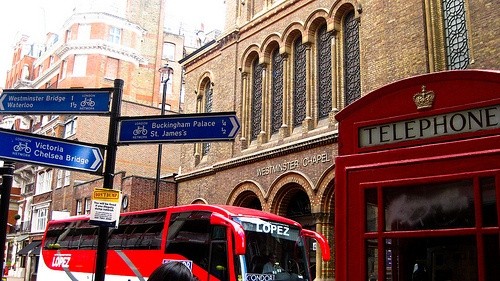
[153,63,174,208]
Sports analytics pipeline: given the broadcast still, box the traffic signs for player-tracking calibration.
[122,111,243,142]
[0,87,111,117]
[1,128,108,175]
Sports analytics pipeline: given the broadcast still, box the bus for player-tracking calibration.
[36,204,332,281]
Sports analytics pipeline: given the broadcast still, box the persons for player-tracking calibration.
[415,255,452,281]
[262,253,284,273]
[148,262,194,281]
[2,260,5,276]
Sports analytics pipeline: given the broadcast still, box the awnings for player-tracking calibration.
[15,235,30,245]
[18,241,41,254]
